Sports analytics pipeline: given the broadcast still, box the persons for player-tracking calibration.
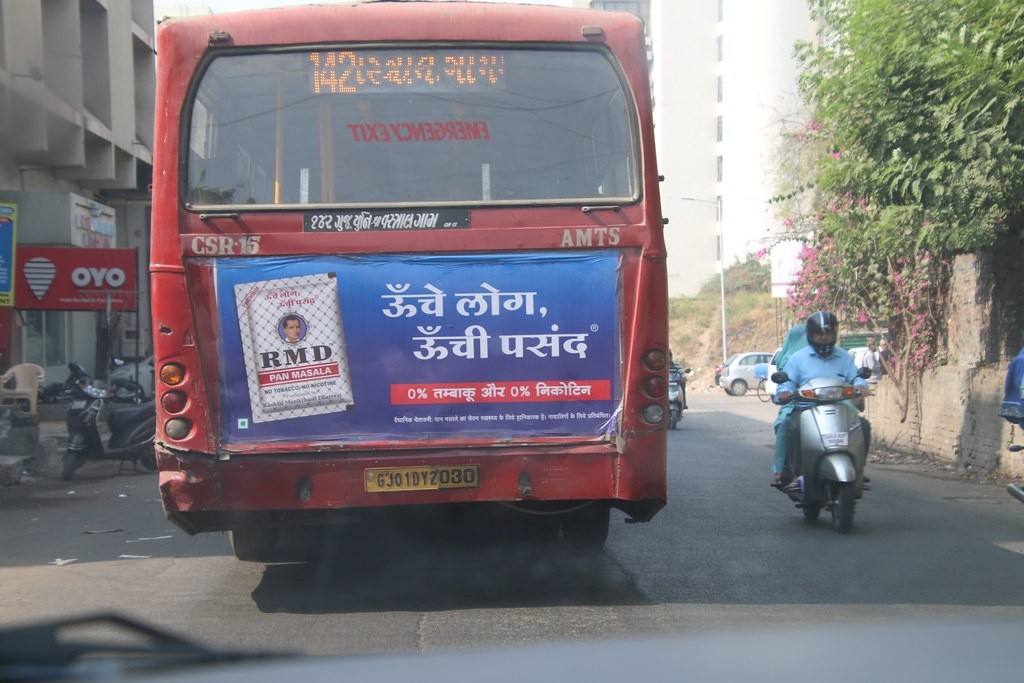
[863,338,894,384]
[669,349,688,409]
[715,363,721,385]
[283,315,301,342]
[755,355,769,365]
[769,311,871,491]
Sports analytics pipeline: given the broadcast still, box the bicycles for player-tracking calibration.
[758,377,772,402]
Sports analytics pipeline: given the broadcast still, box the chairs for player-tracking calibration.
[0,363,45,416]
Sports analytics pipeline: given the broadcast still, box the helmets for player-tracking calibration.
[806,311,838,354]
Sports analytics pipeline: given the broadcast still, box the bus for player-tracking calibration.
[148,1,669,561]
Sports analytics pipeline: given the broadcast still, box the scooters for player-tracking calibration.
[770,365,874,533]
[59,356,157,482]
[667,368,691,431]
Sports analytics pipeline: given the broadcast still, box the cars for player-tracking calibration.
[766,347,783,396]
[715,352,772,397]
[112,355,156,402]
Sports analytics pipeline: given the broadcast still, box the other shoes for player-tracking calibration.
[788,481,801,493]
[684,405,688,409]
[863,484,870,490]
[771,475,784,487]
[862,476,870,482]
